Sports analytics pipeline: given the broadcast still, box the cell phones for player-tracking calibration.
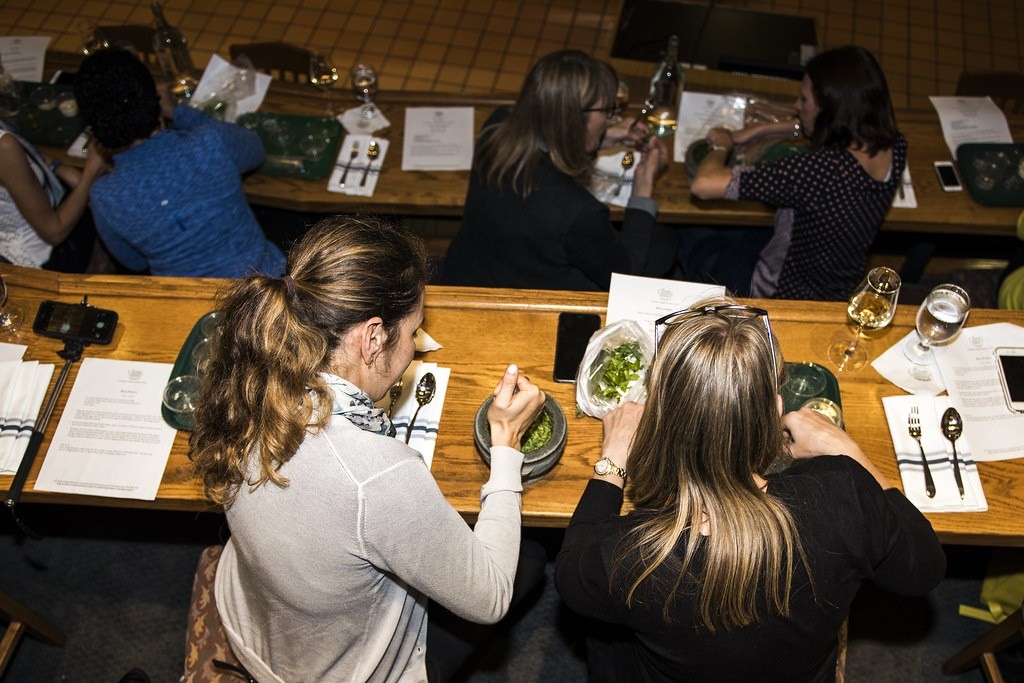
[934,160,963,191]
[553,312,601,383]
[994,346,1024,414]
[32,298,119,345]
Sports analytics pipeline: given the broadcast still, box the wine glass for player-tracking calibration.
[309,47,345,118]
[902,283,971,365]
[351,62,379,120]
[607,73,633,125]
[826,267,901,373]
[0,277,24,335]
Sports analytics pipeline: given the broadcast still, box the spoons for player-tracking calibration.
[941,407,965,499]
[360,141,380,187]
[614,151,635,195]
[406,373,436,445]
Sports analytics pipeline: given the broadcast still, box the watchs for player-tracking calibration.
[594,457,626,480]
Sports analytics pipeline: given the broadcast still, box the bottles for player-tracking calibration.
[643,35,685,138]
[151,3,199,104]
[1,68,21,116]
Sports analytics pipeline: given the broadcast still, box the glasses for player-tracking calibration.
[655,304,779,397]
[581,97,618,119]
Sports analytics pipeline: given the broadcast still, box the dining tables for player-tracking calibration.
[29,77,1024,236]
[0,263,1024,550]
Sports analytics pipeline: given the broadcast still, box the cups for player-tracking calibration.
[782,361,827,411]
[19,85,80,136]
[972,147,1024,191]
[162,313,218,425]
[800,397,843,428]
[238,106,338,160]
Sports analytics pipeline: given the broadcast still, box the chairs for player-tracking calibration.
[184,545,251,683]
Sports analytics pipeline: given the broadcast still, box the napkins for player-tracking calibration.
[890,159,918,208]
[328,135,390,197]
[66,133,88,159]
[335,102,390,135]
[586,144,641,208]
[373,358,451,471]
[881,395,988,513]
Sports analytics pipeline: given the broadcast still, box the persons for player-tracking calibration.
[74,47,287,281]
[188,221,547,683]
[691,46,908,303]
[440,48,669,291]
[553,300,946,683]
[0,127,104,273]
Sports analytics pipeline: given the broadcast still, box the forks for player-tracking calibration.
[908,407,936,498]
[339,141,359,187]
[386,376,403,417]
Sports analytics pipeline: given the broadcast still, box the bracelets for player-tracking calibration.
[710,145,727,154]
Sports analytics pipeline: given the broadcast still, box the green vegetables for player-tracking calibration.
[589,340,646,401]
[521,410,553,454]
